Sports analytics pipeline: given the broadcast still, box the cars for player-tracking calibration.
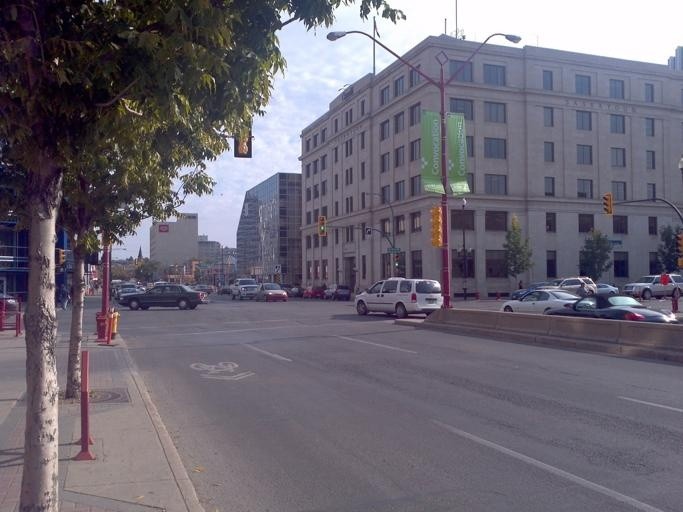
[110,279,213,310]
[281,283,352,301]
[218,285,232,296]
[0,287,18,309]
[499,274,683,324]
[256,283,288,302]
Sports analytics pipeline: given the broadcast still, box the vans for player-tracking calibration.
[354,277,444,318]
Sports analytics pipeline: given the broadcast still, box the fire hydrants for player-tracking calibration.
[496,291,501,300]
[474,291,481,300]
[96,313,109,343]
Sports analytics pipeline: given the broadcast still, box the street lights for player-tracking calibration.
[460,198,470,301]
[326,30,521,309]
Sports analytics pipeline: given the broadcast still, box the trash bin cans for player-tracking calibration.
[111,312,120,340]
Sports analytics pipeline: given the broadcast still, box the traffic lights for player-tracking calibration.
[318,216,326,236]
[393,255,399,272]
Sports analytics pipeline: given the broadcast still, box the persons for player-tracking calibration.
[575,282,588,296]
[671,285,682,312]
[519,279,523,289]
[58,282,70,311]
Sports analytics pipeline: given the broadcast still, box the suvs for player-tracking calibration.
[230,278,260,300]
